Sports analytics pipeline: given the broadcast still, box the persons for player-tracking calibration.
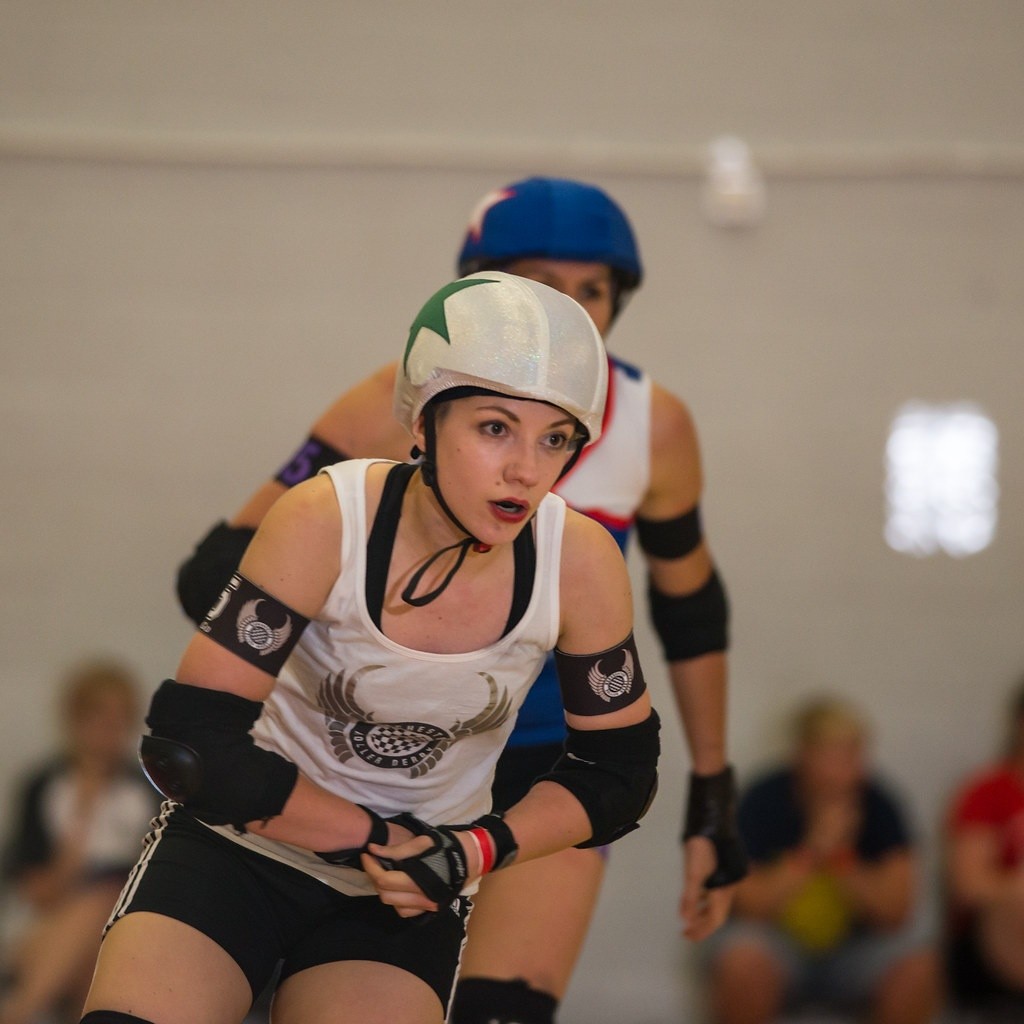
[708,688,1024,1024]
[79,169,751,1024]
[0,658,163,1024]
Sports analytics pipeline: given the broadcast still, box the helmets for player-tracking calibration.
[393,272,608,452]
[457,177,642,288]
